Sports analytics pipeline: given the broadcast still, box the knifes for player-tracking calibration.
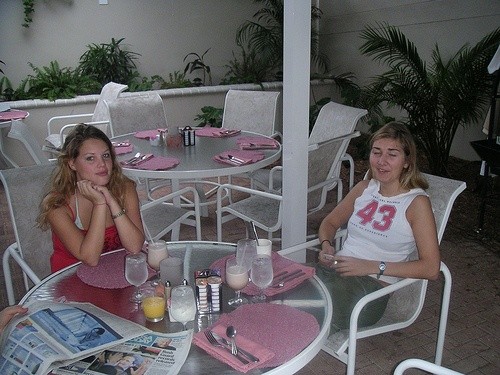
[211,331,259,363]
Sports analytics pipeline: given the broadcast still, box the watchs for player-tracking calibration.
[111,208,127,221]
[377,262,386,279]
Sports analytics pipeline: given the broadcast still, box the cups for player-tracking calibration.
[142,291,166,323]
[160,256,184,287]
[255,239,272,256]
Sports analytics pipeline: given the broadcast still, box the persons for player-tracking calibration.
[0,305,29,329]
[280,121,441,337]
[34,123,146,273]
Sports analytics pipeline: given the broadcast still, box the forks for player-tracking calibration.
[273,272,305,288]
[204,330,249,365]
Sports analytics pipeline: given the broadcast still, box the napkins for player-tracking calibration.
[240,143,277,150]
[111,139,131,146]
[212,129,241,137]
[126,154,157,166]
[192,324,275,374]
[215,154,252,166]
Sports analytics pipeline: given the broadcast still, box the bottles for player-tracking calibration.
[164,281,171,311]
[182,279,188,285]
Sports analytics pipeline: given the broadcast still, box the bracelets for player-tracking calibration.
[319,239,332,246]
[93,202,107,206]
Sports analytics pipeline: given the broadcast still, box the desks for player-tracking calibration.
[109,127,282,241]
[0,108,41,169]
[12,241,333,374]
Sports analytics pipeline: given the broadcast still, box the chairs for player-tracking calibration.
[0,82,500,375]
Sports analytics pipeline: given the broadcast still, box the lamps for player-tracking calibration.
[194,77,202,84]
[4,88,14,98]
[275,71,283,80]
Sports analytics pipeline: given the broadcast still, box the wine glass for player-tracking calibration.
[250,253,273,304]
[225,256,249,308]
[170,285,197,332]
[148,240,168,286]
[235,239,257,284]
[125,252,149,303]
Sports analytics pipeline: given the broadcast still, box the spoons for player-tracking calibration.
[127,152,140,162]
[226,326,238,355]
[227,155,244,162]
